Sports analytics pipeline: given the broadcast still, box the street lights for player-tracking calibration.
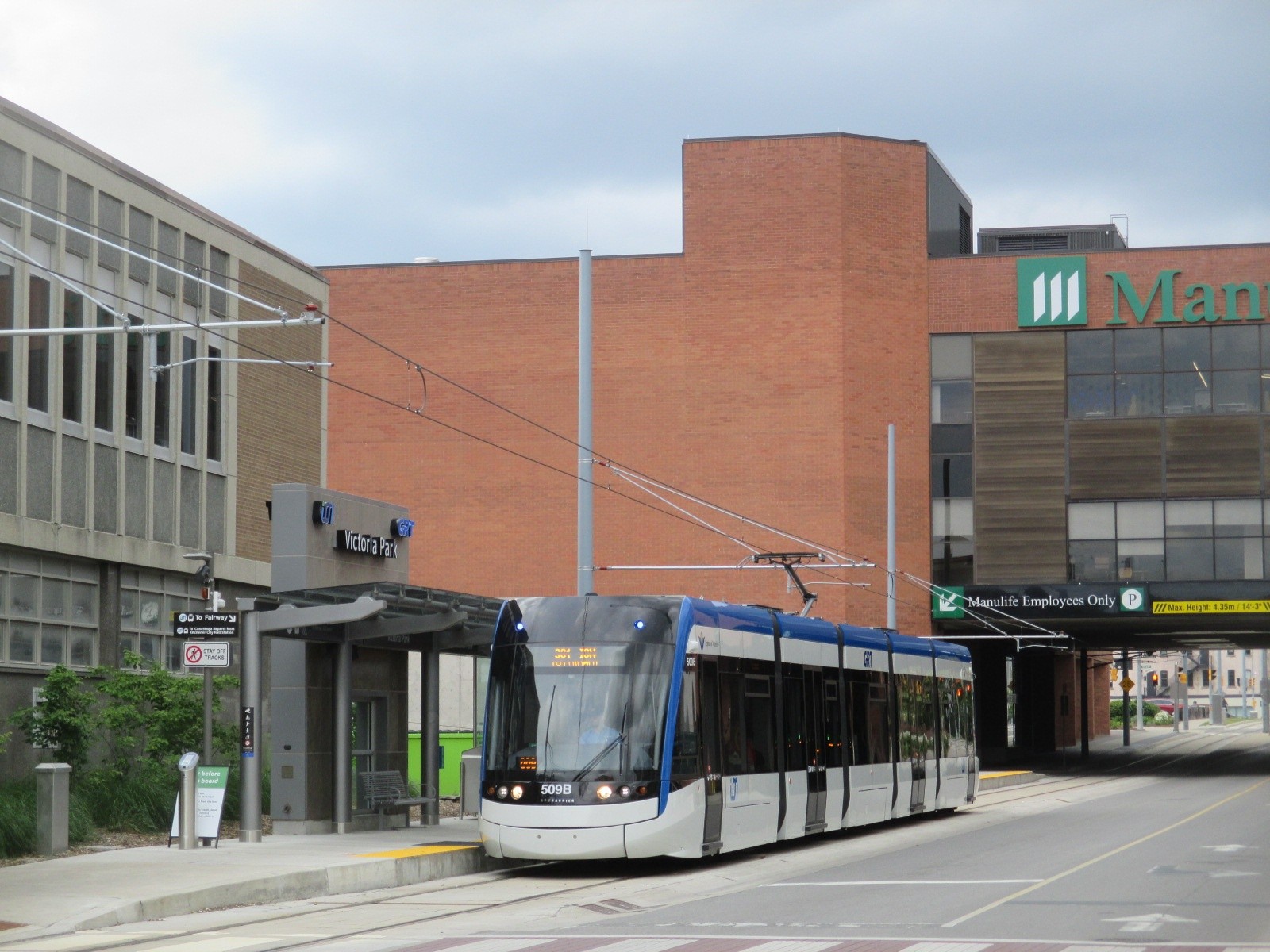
[182,550,216,848]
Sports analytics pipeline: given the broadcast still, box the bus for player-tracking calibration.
[478,550,980,863]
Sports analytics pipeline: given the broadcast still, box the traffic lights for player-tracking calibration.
[1209,670,1216,680]
[1153,674,1158,686]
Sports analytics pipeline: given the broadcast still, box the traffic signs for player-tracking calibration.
[172,612,240,638]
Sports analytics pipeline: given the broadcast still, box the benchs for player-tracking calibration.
[357,769,438,830]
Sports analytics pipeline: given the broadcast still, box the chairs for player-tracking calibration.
[1092,556,1110,581]
[1139,560,1165,581]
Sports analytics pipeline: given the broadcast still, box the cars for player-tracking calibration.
[1143,698,1183,721]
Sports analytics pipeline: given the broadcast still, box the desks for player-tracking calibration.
[1107,569,1148,580]
[1085,411,1107,417]
[1164,405,1195,414]
[1217,401,1247,413]
